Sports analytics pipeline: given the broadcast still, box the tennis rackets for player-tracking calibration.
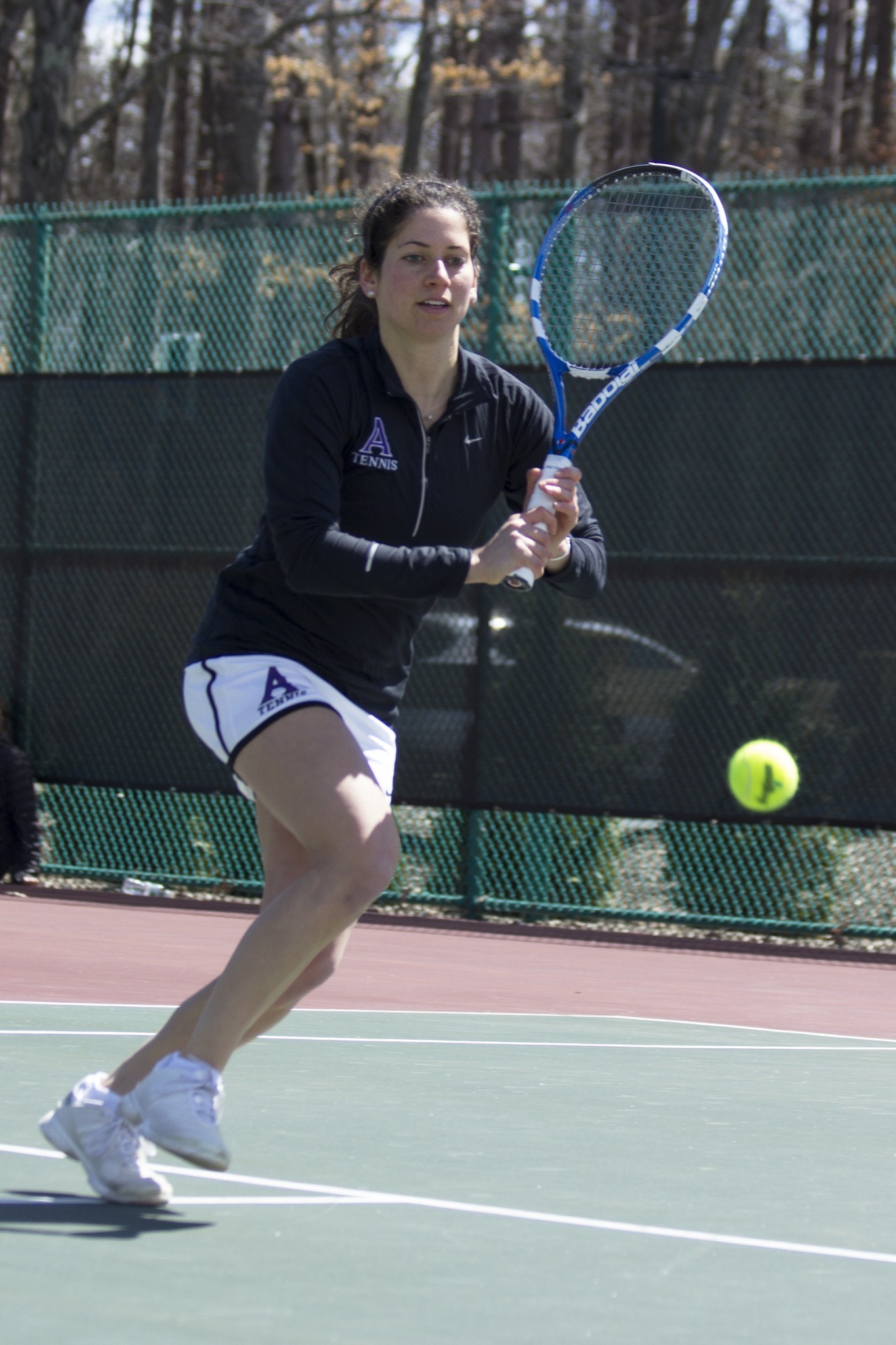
[502,156,733,594]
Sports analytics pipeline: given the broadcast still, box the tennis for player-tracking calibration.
[727,738,803,813]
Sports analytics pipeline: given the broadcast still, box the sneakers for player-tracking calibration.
[116,1052,231,1172]
[39,1072,172,1205]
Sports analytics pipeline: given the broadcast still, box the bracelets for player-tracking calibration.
[550,536,570,561]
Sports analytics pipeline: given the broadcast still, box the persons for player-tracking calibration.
[40,175,606,1206]
[0,698,40,883]
